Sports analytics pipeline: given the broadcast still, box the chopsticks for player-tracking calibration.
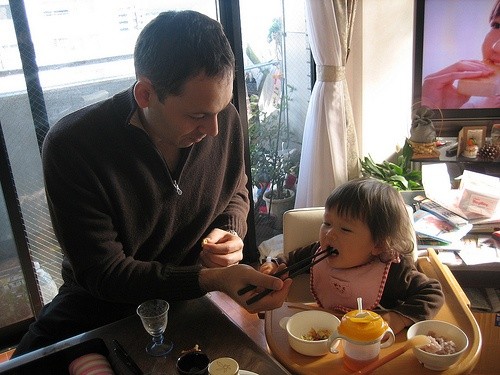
[237,245,335,304]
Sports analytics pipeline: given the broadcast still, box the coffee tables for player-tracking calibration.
[396,191,500,274]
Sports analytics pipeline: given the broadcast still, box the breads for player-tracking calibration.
[456,60,500,97]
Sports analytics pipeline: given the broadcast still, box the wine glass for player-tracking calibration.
[134,297,173,358]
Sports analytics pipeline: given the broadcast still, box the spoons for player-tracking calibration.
[359,334,429,375]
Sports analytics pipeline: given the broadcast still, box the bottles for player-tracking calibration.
[327,308,396,372]
[208,357,240,375]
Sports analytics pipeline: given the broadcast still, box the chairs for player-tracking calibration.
[264,205,483,375]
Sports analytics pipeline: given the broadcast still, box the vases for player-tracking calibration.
[263,188,295,229]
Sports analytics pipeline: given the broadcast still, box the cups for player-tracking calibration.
[176,349,209,375]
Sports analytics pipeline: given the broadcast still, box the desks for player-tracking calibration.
[0,296,291,374]
[409,136,500,179]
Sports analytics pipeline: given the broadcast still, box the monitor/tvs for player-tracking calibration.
[411,0,500,157]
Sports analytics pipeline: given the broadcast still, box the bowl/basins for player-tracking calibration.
[407,319,469,371]
[279,309,341,358]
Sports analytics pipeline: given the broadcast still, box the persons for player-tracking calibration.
[11,10,293,363]
[259,177,444,341]
[420,0,500,109]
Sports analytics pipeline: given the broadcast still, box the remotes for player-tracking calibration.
[420,198,468,228]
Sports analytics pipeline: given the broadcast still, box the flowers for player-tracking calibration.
[249,94,298,211]
